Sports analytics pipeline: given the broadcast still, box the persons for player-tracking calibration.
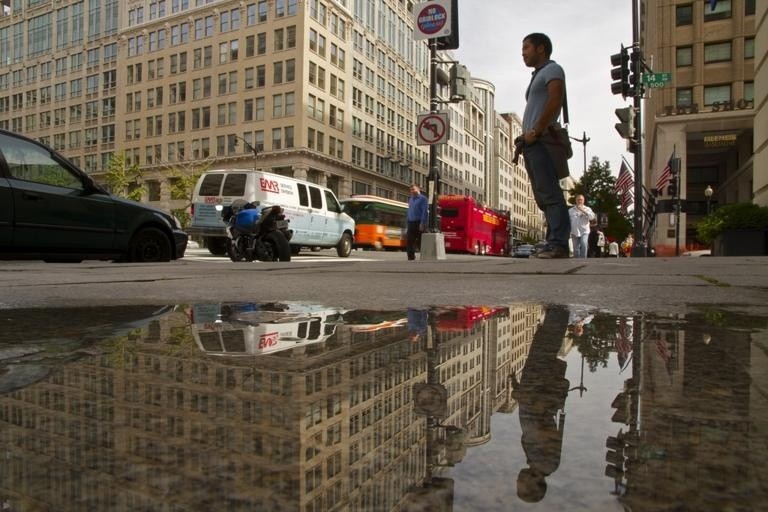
[506,305,570,504]
[406,184,428,261]
[511,33,574,258]
[568,194,595,256]
[587,226,634,257]
[567,311,596,336]
[405,309,428,342]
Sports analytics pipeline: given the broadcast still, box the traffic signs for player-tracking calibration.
[639,70,672,89]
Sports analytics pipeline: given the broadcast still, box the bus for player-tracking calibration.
[341,309,410,336]
[436,192,513,257]
[428,307,512,336]
[336,191,410,252]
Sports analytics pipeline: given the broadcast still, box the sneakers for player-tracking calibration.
[529,245,569,259]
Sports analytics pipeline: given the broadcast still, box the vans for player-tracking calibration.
[186,164,358,260]
[183,301,344,362]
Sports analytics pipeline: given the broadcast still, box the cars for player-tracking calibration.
[513,242,535,258]
[677,248,711,257]
[0,304,177,399]
[0,127,194,265]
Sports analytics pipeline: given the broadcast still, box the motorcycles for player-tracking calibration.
[206,301,292,333]
[212,196,295,264]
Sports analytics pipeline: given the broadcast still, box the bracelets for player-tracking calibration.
[528,126,539,137]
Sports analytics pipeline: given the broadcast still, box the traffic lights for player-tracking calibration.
[599,428,626,488]
[449,65,469,97]
[614,106,636,140]
[609,41,629,103]
[607,388,630,428]
[627,46,645,99]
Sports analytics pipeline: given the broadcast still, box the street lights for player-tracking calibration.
[703,182,715,224]
[232,134,258,170]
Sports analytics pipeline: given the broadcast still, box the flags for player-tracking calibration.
[614,160,633,215]
[656,154,673,190]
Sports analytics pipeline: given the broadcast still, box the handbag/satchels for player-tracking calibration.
[547,378,569,409]
[552,154,568,178]
[551,128,572,160]
[549,359,568,378]
